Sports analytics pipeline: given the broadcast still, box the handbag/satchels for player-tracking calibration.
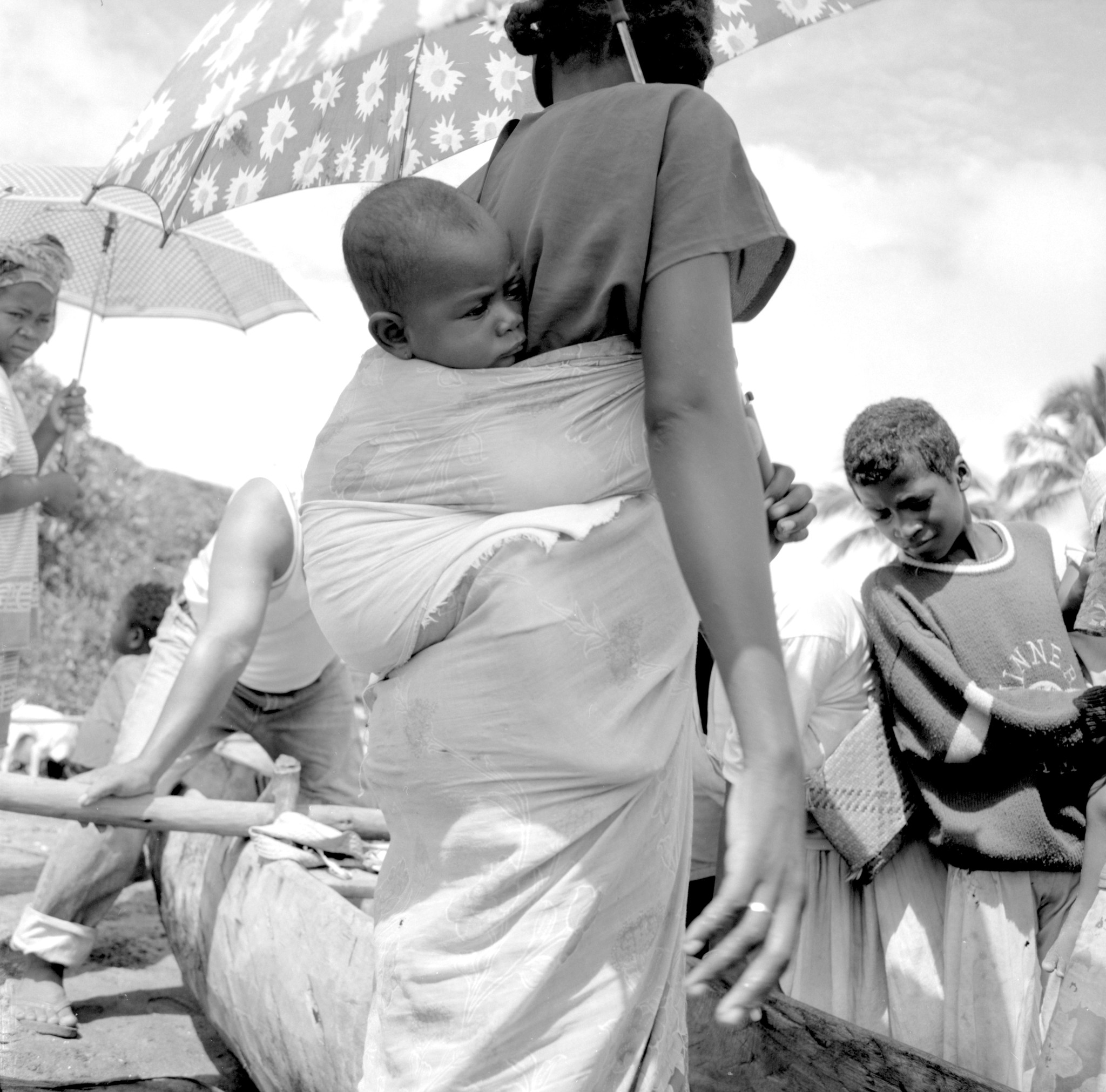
[809,697,919,887]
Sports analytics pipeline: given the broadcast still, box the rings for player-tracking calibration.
[745,897,772,917]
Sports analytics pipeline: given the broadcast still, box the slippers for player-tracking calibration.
[10,995,79,1039]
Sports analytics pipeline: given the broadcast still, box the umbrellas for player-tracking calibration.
[1,165,318,511]
[88,0,877,520]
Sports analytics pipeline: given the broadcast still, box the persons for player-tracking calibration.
[356,0,822,1090]
[68,584,179,766]
[0,234,86,781]
[686,581,948,1063]
[0,473,375,1041]
[845,395,1106,981]
[303,175,605,674]
